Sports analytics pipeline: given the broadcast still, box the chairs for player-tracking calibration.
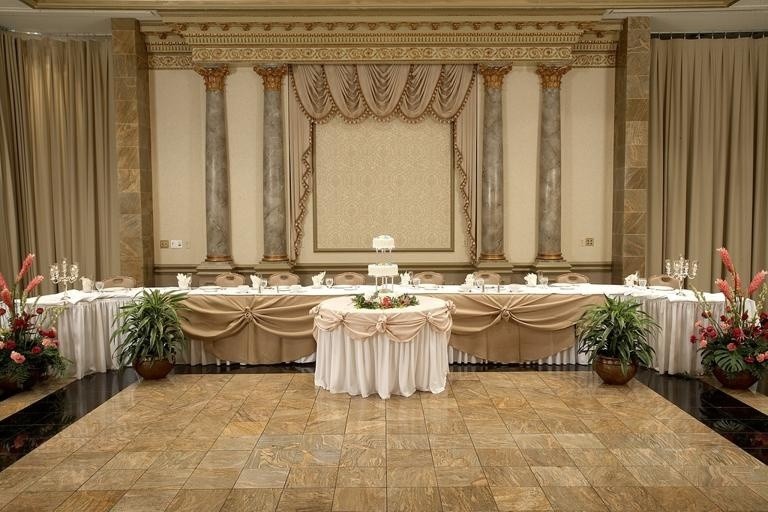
[556,274,591,282]
[649,275,678,287]
[217,274,246,285]
[268,274,302,286]
[101,277,137,287]
[333,273,366,285]
[415,271,445,284]
[474,272,502,284]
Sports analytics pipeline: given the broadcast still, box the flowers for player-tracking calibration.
[0,253,67,383]
[687,247,768,363]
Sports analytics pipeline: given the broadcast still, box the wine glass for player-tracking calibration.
[95,282,105,298]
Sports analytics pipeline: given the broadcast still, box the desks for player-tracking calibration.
[22,282,753,372]
[311,290,456,396]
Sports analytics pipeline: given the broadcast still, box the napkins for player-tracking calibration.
[465,274,475,286]
[400,272,410,284]
[625,274,638,286]
[177,273,191,283]
[312,271,327,287]
[525,273,537,286]
[250,274,261,286]
[79,277,90,289]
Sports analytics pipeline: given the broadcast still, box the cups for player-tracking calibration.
[412,279,421,287]
[259,279,268,289]
[476,278,484,286]
[325,278,333,288]
[540,276,549,286]
[638,278,647,287]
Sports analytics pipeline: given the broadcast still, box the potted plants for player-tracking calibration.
[576,294,658,382]
[107,286,193,378]
